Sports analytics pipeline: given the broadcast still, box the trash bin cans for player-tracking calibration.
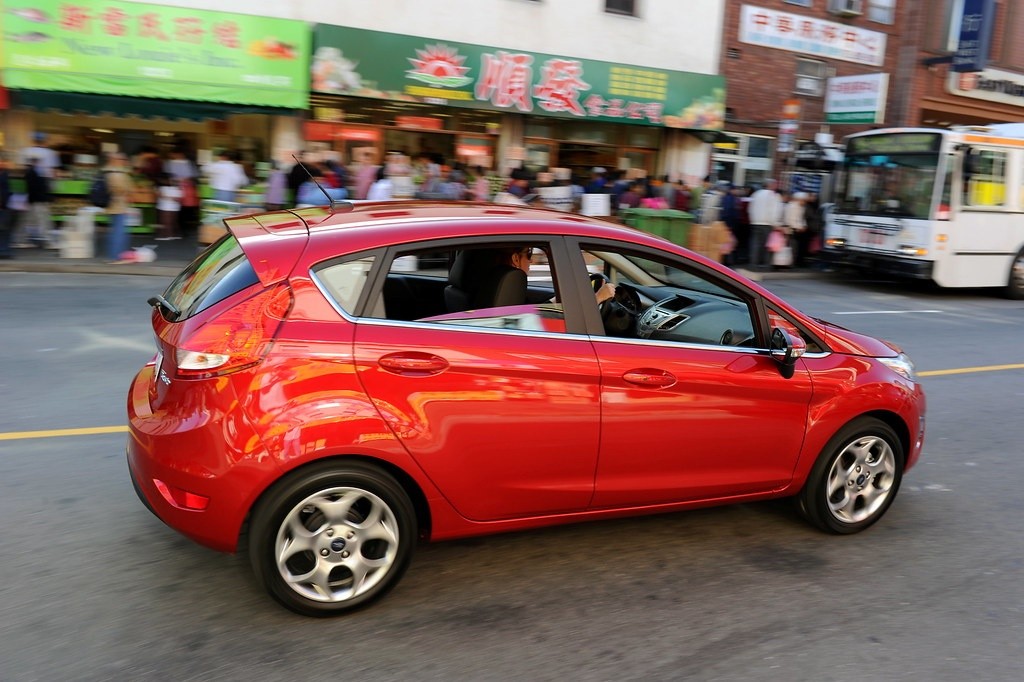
[617,206,695,251]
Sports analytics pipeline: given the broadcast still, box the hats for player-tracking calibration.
[593,167,607,173]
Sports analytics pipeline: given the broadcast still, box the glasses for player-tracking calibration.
[519,248,532,260]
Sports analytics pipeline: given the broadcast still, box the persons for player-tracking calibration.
[491,243,616,309]
[0,128,825,265]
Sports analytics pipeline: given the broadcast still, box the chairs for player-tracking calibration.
[473,262,527,308]
[444,248,480,311]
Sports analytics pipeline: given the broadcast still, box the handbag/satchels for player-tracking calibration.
[767,230,785,252]
[770,246,793,265]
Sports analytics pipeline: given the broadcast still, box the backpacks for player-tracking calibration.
[90,166,113,208]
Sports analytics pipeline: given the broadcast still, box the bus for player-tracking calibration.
[817,125,1023,300]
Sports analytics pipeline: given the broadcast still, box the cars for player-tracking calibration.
[124,199,926,618]
